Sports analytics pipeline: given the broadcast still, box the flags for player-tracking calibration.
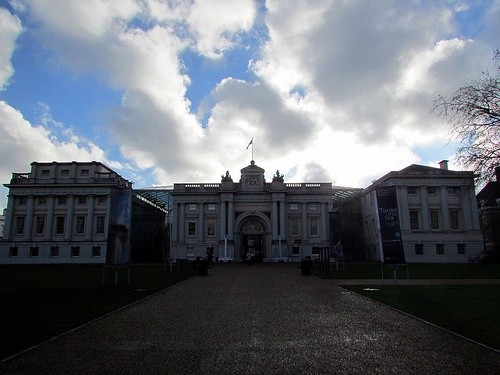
[246,140,252,149]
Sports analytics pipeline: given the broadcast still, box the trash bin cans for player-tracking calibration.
[196,256,209,276]
[301,256,312,276]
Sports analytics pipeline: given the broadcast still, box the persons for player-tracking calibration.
[276,170,279,178]
[226,171,229,177]
